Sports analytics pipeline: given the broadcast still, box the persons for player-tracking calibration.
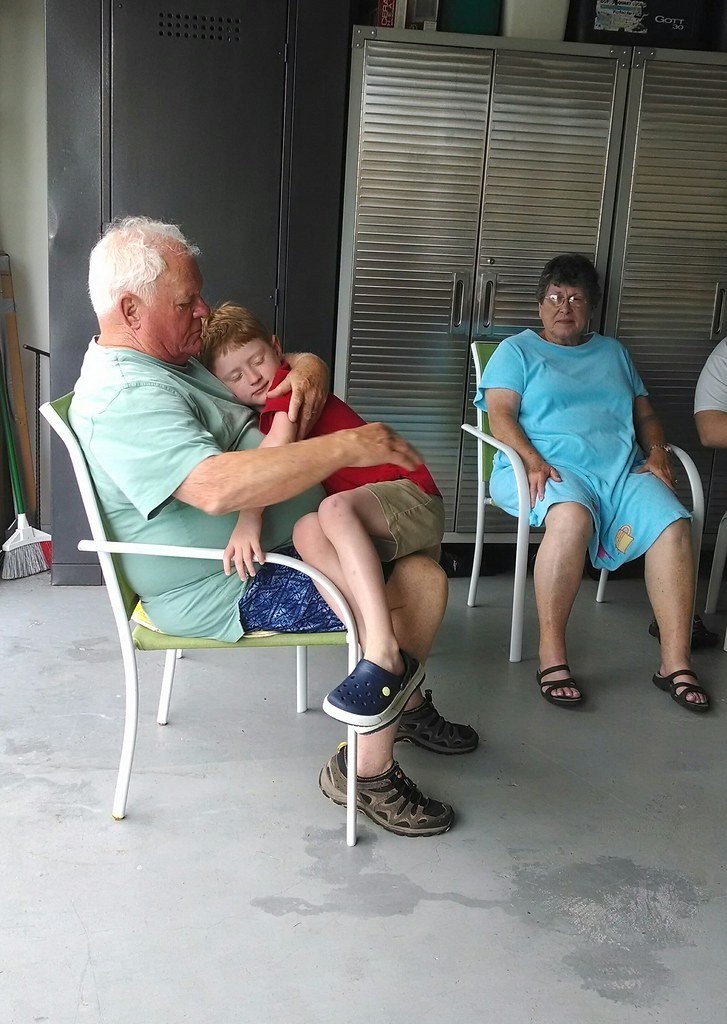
[67,214,478,837]
[474,255,711,713]
[199,301,445,736]
[695,336,727,449]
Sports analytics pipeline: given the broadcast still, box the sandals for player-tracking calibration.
[535,664,581,707]
[321,649,428,735]
[651,668,712,714]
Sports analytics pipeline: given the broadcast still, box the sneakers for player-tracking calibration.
[319,744,458,838]
[390,700,479,754]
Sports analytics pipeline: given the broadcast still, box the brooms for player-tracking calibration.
[0,350,54,580]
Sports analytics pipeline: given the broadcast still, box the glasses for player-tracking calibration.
[544,294,587,306]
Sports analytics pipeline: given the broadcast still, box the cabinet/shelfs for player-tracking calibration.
[331,24,727,542]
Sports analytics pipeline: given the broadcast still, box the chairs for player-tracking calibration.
[459,342,706,663]
[41,389,362,846]
